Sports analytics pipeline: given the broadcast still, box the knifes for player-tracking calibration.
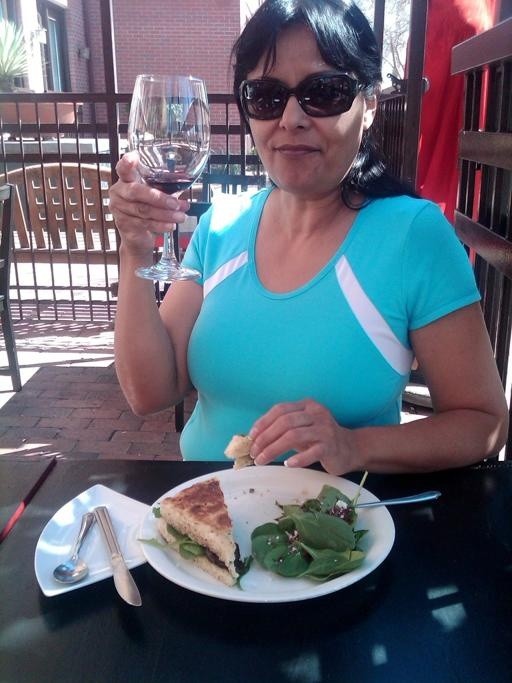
[97,506,140,607]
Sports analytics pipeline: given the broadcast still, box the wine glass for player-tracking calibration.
[124,72,211,282]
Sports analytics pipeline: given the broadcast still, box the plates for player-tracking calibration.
[32,484,148,600]
[147,464,400,605]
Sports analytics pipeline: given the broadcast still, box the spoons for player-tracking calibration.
[54,510,97,583]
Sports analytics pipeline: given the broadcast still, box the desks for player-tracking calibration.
[2,460,58,540]
[3,460,511,682]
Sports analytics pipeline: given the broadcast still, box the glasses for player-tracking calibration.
[239,74,373,119]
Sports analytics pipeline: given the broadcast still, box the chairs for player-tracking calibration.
[164,170,266,433]
[0,184,22,392]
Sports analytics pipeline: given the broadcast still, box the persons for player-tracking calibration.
[108,1,511,478]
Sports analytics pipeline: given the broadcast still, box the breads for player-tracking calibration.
[157,478,241,587]
[224,434,257,470]
